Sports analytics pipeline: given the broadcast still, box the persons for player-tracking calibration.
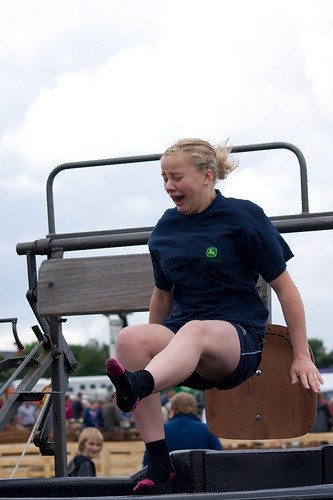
[0,385,207,434]
[141,391,222,473]
[67,426,103,479]
[311,390,333,433]
[104,138,325,492]
